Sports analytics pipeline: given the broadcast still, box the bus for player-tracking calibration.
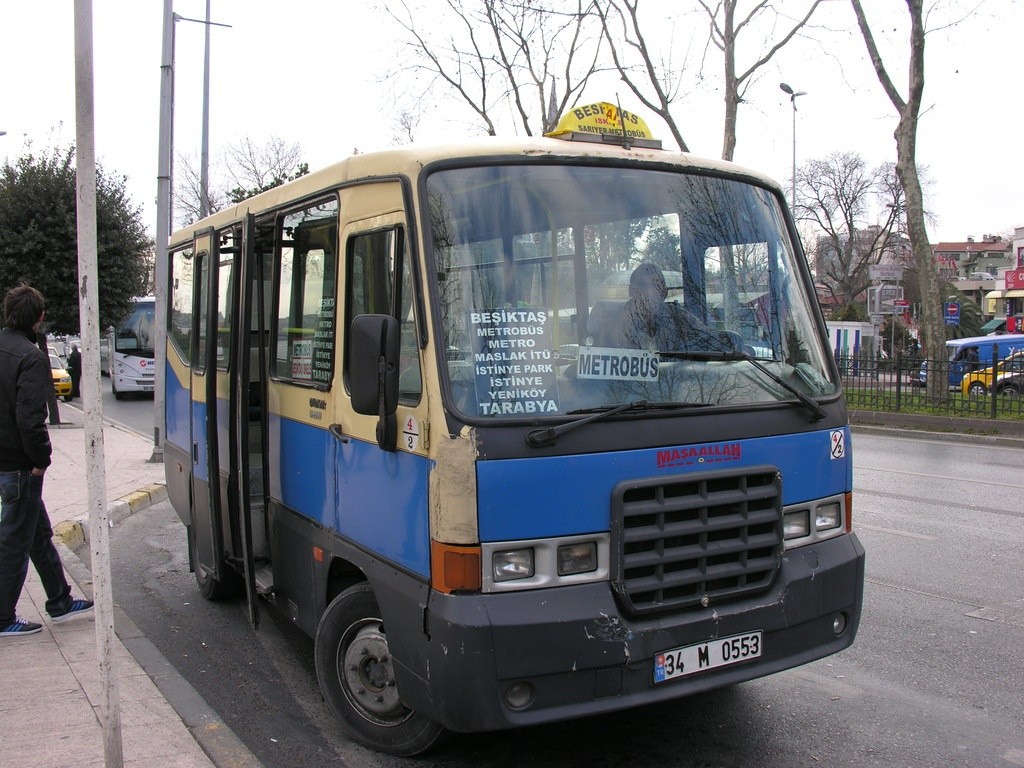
[163,100,865,760]
[108,297,157,400]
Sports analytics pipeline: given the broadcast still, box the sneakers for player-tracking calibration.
[0,617,43,637]
[50,597,95,622]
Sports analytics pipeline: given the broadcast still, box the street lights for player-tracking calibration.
[887,203,908,370]
[780,83,807,219]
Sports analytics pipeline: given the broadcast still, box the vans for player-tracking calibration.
[919,335,1024,386]
[970,273,994,281]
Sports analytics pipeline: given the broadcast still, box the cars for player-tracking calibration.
[961,352,1024,398]
[989,371,1023,398]
[48,347,59,356]
[65,340,82,367]
[48,354,72,401]
[100,339,110,374]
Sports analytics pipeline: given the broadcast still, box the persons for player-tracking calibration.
[68,344,82,398]
[600,264,712,352]
[0,286,95,637]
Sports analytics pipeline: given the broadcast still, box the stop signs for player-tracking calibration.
[946,304,958,315]
